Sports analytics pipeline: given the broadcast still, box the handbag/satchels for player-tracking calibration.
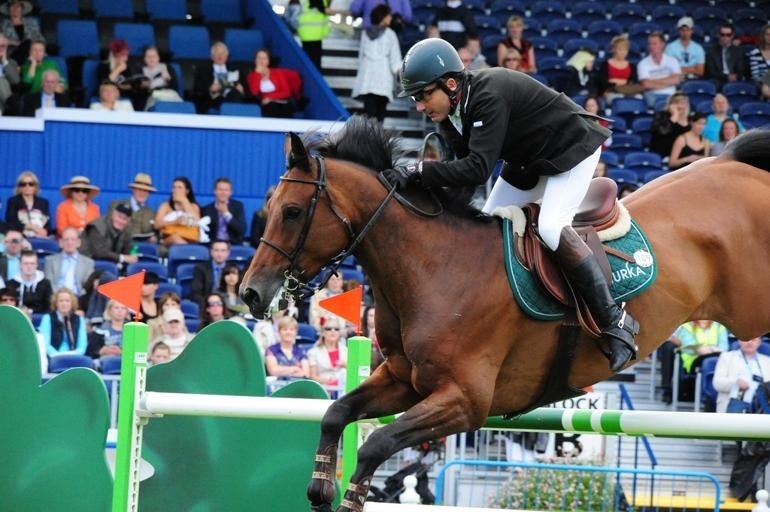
[727,390,752,415]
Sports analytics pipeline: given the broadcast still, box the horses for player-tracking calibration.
[236,118,770,511]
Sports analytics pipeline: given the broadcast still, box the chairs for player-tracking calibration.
[656,327,770,503]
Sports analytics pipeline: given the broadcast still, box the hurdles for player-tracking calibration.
[112,321,768,511]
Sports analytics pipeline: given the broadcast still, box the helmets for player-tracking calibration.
[397,37,465,97]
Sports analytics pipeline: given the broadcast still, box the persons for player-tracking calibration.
[1,170,380,400]
[289,1,535,124]
[675,317,770,454]
[1,1,301,116]
[560,17,770,201]
[394,37,639,371]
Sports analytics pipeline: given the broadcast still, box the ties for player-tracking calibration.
[47,96,52,107]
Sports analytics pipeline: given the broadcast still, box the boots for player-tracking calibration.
[560,254,640,372]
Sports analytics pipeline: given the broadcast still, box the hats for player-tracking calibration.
[127,172,157,192]
[677,16,694,29]
[0,0,33,15]
[61,176,99,200]
[163,308,184,322]
[116,203,133,216]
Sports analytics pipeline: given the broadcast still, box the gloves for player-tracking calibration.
[383,163,421,190]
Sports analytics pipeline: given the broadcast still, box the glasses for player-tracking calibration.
[69,187,93,193]
[410,85,441,102]
[19,181,36,187]
[719,32,732,36]
[322,326,342,331]
[208,302,222,306]
[72,188,90,193]
